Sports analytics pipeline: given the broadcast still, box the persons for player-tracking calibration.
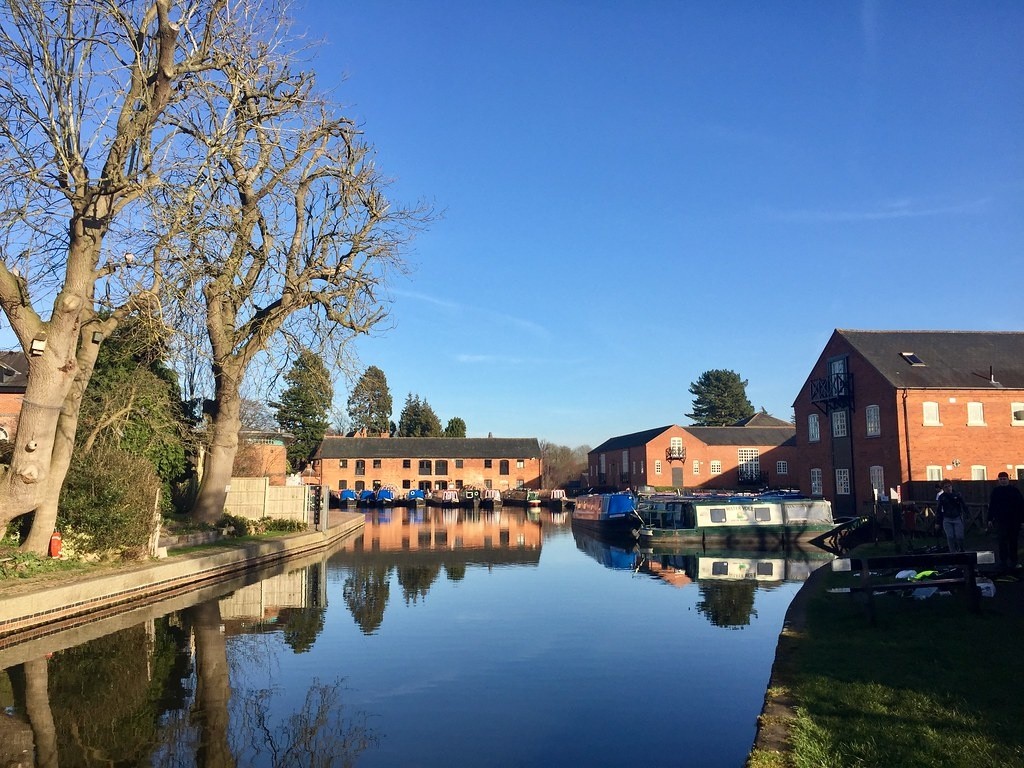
[987,472,1024,575]
[935,479,971,553]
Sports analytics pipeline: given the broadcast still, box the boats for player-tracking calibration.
[573,532,635,572]
[631,492,834,541]
[337,487,567,506]
[632,542,835,584]
[573,491,636,532]
